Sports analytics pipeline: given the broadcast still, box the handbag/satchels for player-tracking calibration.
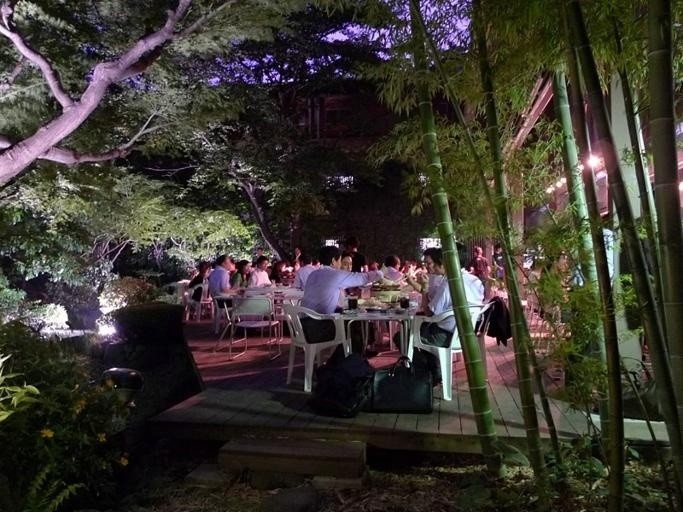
[370,356,434,415]
[309,374,370,418]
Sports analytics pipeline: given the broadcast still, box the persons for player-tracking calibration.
[413,249,486,393]
[299,245,386,368]
[468,246,493,338]
[491,243,505,282]
[187,248,447,360]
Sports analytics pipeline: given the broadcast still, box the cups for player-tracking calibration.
[342,284,420,316]
